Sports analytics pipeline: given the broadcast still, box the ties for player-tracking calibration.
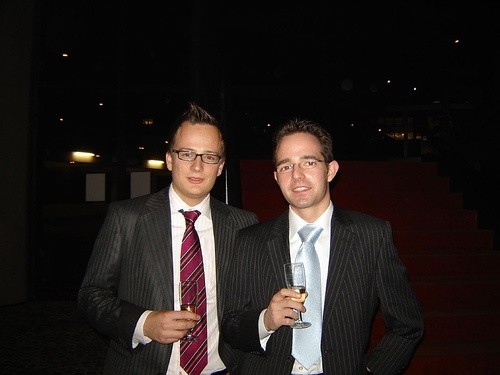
[178,208,208,375]
[292,224,324,369]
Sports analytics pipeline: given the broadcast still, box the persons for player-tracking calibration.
[219,118,425,375]
[77,101,261,375]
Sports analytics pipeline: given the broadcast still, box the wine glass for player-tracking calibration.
[284,263,311,329]
[180,281,200,342]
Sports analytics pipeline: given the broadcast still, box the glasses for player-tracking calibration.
[172,149,222,165]
[275,160,325,174]
